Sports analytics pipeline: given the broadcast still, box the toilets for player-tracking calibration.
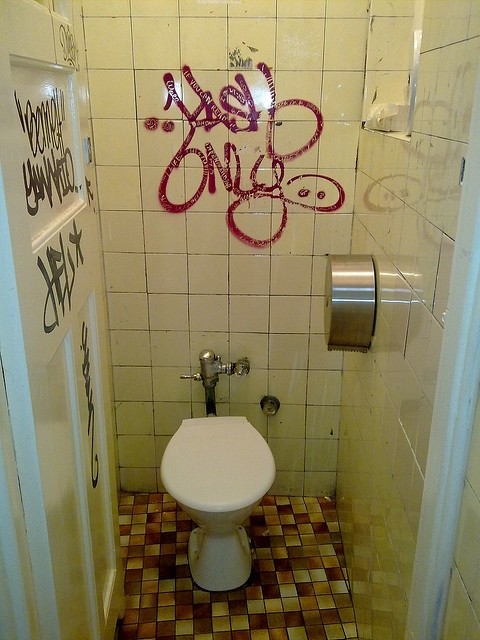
[159,415,278,593]
